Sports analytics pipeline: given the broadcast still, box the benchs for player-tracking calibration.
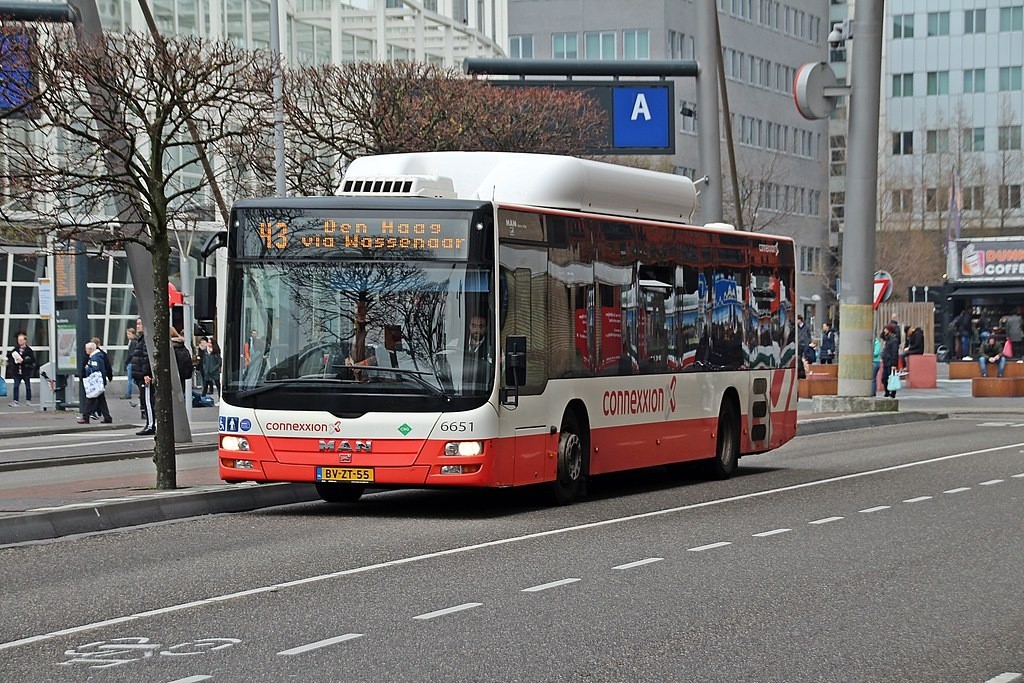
[949,361,1024,380]
[798,365,837,399]
[972,376,1024,398]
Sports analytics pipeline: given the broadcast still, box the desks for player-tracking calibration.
[908,353,937,388]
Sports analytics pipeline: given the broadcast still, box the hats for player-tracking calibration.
[885,325,894,332]
[904,326,911,332]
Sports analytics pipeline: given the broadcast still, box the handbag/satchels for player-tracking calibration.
[887,369,900,391]
[83,368,105,398]
[1002,337,1013,357]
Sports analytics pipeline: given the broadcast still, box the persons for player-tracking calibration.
[979,335,1005,378]
[170,327,222,406]
[819,322,836,364]
[797,315,818,400]
[120,318,156,435]
[242,330,276,380]
[900,326,924,371]
[78,338,113,423]
[9,334,36,407]
[873,314,901,398]
[619,317,796,373]
[341,294,387,375]
[428,313,505,375]
[954,309,1024,360]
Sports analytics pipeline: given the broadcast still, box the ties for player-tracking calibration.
[469,342,480,352]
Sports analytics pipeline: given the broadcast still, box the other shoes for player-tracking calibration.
[76,419,89,424]
[25,400,31,405]
[898,370,909,376]
[9,400,19,406]
[101,420,111,423]
[136,429,156,435]
[998,374,1002,377]
[118,394,131,399]
[983,373,988,377]
[962,355,973,361]
[90,414,99,420]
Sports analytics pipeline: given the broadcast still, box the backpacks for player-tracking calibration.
[174,345,194,379]
[947,319,959,336]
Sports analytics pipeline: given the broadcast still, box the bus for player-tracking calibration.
[194,147,801,506]
